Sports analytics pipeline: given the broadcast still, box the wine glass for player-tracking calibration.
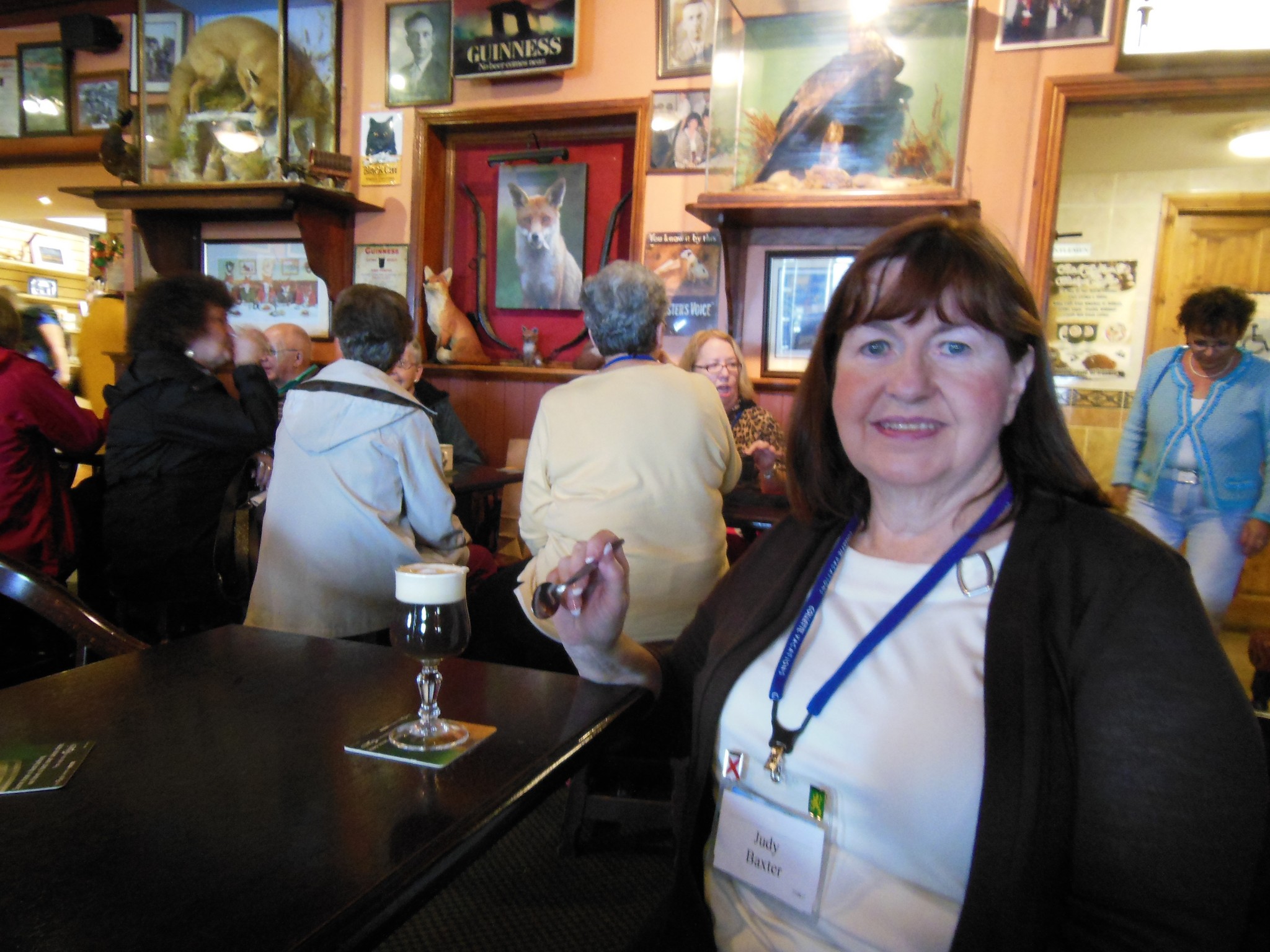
[388,562,471,751]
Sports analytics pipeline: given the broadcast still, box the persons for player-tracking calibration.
[1,286,79,490]
[1112,285,1270,637]
[390,338,490,543]
[144,34,176,83]
[221,260,317,310]
[243,283,500,662]
[259,323,323,452]
[1013,0,1100,37]
[102,274,274,644]
[394,10,449,102]
[0,291,109,690]
[670,0,712,67]
[673,92,725,169]
[79,88,110,129]
[679,328,789,564]
[515,258,743,668]
[548,222,1270,952]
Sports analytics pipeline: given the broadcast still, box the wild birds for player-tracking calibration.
[753,11,905,182]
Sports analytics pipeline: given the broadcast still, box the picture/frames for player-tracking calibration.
[761,244,861,382]
[996,0,1114,51]
[384,1,456,108]
[655,0,718,78]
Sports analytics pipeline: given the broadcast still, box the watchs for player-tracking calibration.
[762,462,776,479]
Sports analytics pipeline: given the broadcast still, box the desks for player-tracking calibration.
[2,621,676,952]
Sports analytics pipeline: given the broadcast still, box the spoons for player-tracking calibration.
[532,538,625,619]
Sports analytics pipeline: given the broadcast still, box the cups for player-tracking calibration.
[1057,322,1097,343]
[440,444,453,470]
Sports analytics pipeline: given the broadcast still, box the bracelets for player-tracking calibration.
[253,448,271,456]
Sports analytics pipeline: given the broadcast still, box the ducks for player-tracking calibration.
[99,109,137,187]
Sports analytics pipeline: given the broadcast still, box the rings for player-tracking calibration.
[266,464,273,472]
[257,461,266,467]
